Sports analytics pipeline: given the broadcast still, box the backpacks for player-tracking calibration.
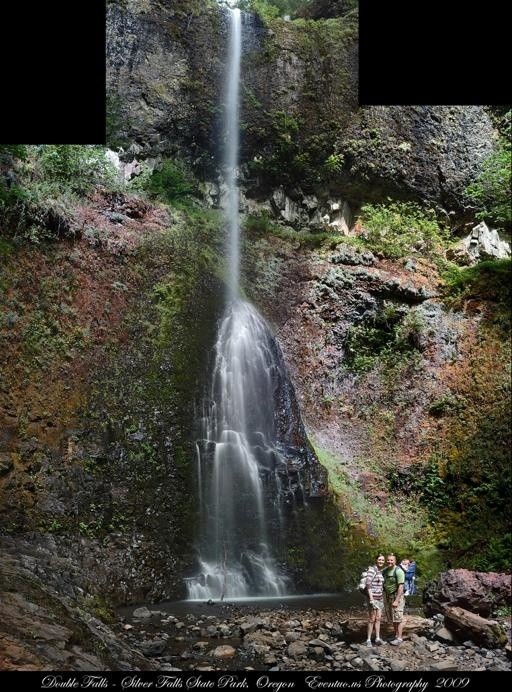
[358,565,377,594]
[383,559,417,597]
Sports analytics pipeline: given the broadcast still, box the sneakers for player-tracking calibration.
[365,638,372,647]
[390,638,403,645]
[375,638,387,645]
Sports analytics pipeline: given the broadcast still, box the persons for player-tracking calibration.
[382,553,406,646]
[364,554,386,649]
